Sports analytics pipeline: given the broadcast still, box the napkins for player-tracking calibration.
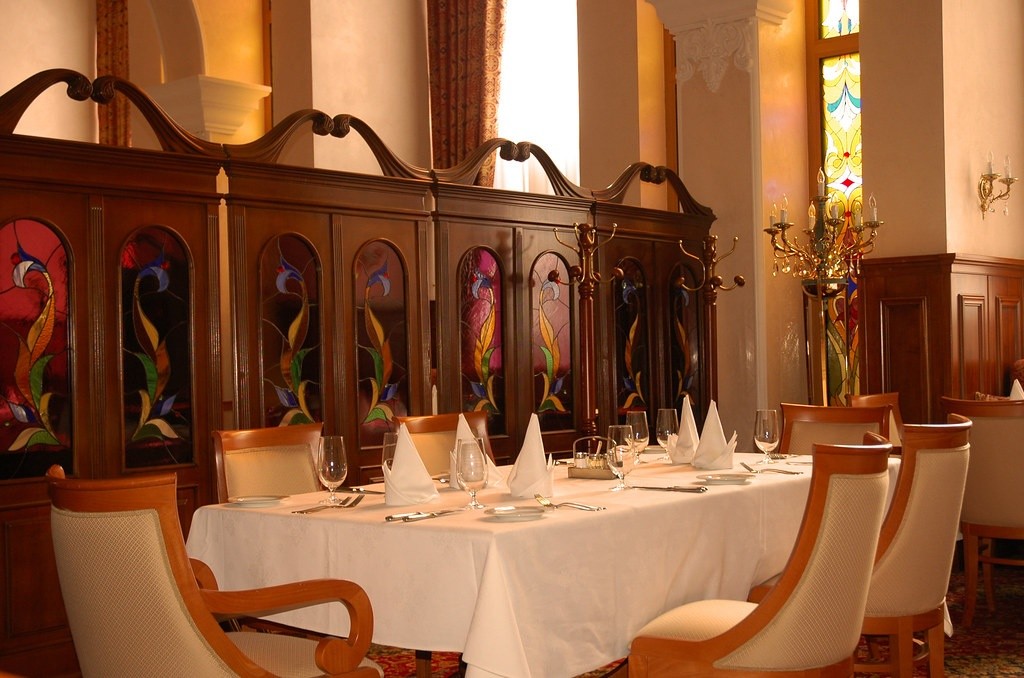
[1010,379,1024,401]
[692,400,737,468]
[382,421,438,505]
[666,395,701,465]
[450,412,504,489]
[505,411,556,498]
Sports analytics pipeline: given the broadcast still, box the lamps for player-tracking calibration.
[979,151,1018,220]
[765,167,883,287]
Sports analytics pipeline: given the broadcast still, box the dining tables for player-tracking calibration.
[185,452,899,678]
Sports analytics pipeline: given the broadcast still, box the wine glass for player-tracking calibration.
[624,410,650,465]
[656,408,679,464]
[457,438,488,509]
[753,408,779,465]
[607,425,636,491]
[382,433,401,471]
[318,436,348,503]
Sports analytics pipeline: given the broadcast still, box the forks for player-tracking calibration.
[292,495,364,514]
[534,494,600,511]
[740,462,804,476]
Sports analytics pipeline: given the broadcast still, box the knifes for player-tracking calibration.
[385,509,465,522]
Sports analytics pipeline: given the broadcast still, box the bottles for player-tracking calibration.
[574,452,615,470]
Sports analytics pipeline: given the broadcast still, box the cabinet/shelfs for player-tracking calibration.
[0,132,223,678]
[592,202,717,453]
[226,161,438,489]
[438,182,595,466]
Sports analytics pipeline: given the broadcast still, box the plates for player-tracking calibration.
[696,474,754,484]
[642,445,668,454]
[483,506,545,519]
[228,494,290,507]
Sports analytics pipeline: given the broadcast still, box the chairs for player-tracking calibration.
[942,396,1023,625]
[846,393,906,446]
[47,465,385,678]
[747,413,971,677]
[211,422,325,503]
[778,402,894,455]
[627,432,893,677]
[393,409,494,477]
